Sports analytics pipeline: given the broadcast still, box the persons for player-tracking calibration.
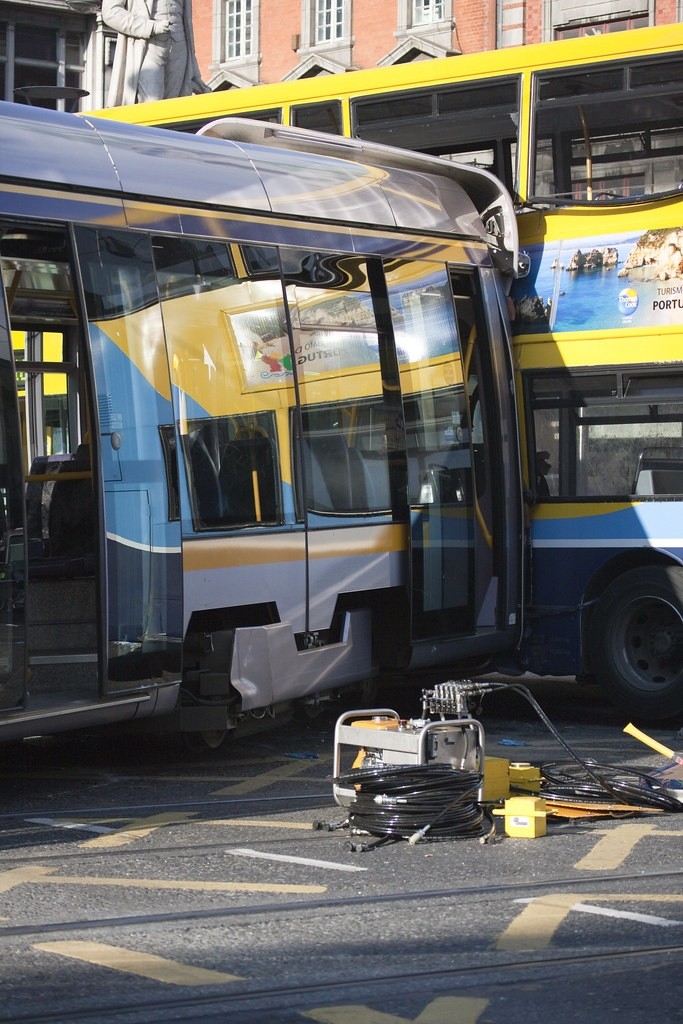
[101,0,212,108]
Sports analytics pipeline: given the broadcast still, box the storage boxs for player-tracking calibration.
[476,755,511,807]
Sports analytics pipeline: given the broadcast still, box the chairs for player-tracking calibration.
[631,447,683,495]
[49,425,378,642]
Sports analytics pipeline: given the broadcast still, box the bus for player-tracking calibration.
[9,23,683,725]
[0,103,527,745]
